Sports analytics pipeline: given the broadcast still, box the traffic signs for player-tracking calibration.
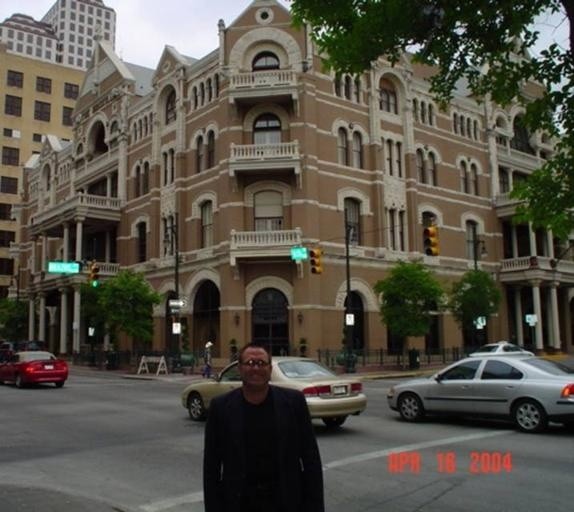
[289,246,307,262]
[47,261,79,274]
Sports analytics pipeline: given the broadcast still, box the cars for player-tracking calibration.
[181,355,367,430]
[0,338,68,388]
[384,338,574,434]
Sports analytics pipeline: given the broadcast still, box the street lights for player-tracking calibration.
[466,226,489,270]
[336,204,361,374]
[159,212,184,373]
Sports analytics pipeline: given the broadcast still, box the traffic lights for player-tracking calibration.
[89,263,101,289]
[308,248,323,277]
[422,224,441,259]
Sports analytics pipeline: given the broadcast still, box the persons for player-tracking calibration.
[201,341,214,378]
[229,346,238,363]
[203,341,325,512]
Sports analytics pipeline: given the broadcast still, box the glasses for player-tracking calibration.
[239,359,268,367]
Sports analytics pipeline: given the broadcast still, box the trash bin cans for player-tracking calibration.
[107,352,119,371]
[409,350,420,370]
